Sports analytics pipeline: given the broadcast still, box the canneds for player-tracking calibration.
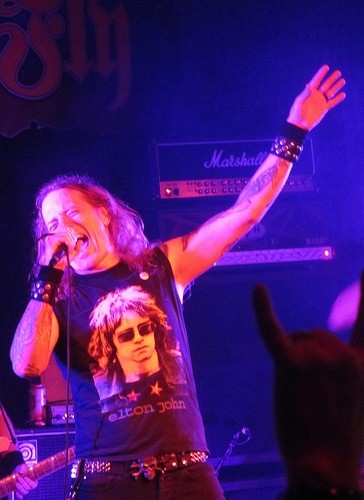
[29,384,46,426]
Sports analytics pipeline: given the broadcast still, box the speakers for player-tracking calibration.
[182,257,330,422]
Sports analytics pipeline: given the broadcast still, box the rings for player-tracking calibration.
[323,92,329,101]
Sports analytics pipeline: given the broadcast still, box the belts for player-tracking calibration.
[72,449,209,480]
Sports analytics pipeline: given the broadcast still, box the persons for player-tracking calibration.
[87,285,187,399]
[0,404,38,500]
[10,65,345,500]
[255,279,364,500]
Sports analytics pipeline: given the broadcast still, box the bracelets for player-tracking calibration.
[33,265,64,285]
[277,121,309,145]
[270,135,304,162]
[29,280,54,303]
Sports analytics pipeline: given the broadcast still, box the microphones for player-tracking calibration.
[49,233,81,267]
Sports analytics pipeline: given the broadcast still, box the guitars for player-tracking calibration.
[0,445,76,500]
